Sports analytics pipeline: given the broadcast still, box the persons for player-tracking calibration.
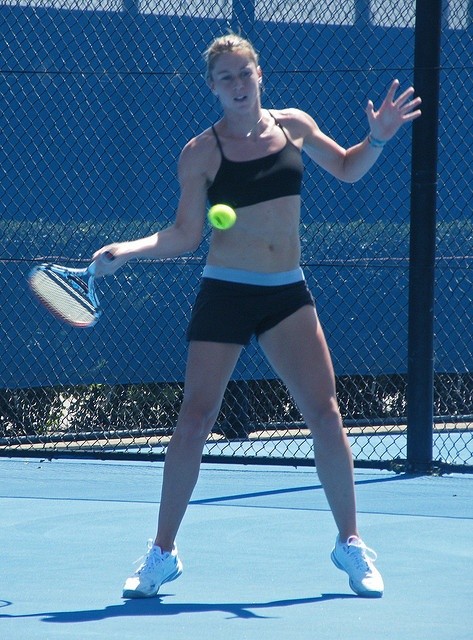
[90,34,423,598]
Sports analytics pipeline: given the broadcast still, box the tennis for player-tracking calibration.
[208,204,237,229]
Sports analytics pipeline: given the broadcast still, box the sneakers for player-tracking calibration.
[331,532,384,598]
[122,539,183,599]
